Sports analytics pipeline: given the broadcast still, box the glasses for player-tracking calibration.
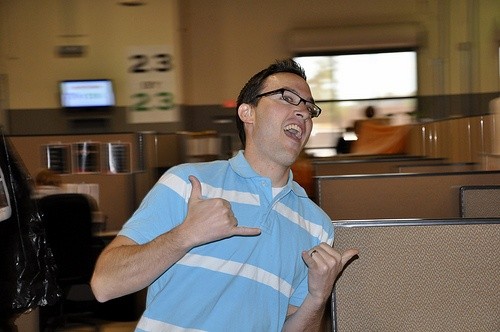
[247,87,322,118]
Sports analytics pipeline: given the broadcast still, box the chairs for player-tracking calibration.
[0,135,148,332]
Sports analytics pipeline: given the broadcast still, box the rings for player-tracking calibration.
[310,249,318,257]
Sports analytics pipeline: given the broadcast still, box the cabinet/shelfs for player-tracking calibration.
[6,131,247,236]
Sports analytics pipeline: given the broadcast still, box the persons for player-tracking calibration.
[90,57,360,332]
